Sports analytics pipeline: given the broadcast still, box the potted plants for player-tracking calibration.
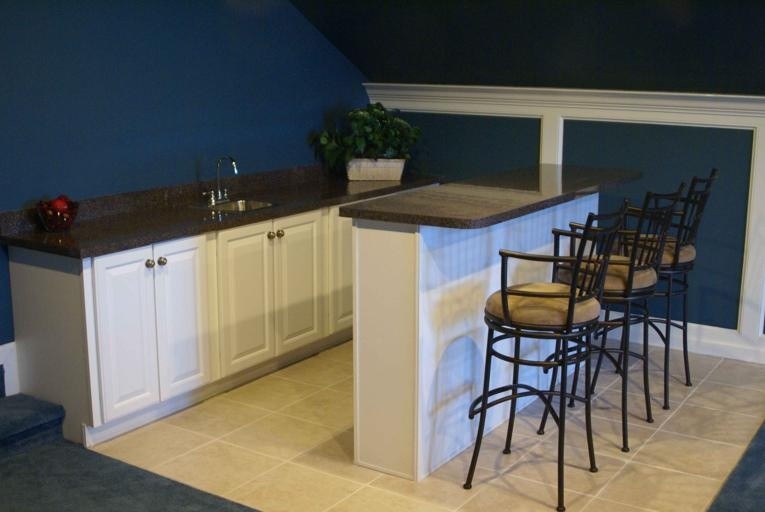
[307,99,424,183]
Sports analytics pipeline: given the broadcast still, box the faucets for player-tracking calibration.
[215,155,239,200]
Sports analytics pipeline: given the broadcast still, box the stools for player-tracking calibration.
[535,181,689,453]
[464,198,631,509]
[591,167,724,411]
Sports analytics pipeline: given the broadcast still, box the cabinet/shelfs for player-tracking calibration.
[330,201,353,348]
[6,231,218,451]
[218,208,330,392]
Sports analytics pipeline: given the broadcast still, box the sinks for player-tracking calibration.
[213,196,275,212]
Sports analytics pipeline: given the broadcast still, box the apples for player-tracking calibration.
[36,196,72,229]
